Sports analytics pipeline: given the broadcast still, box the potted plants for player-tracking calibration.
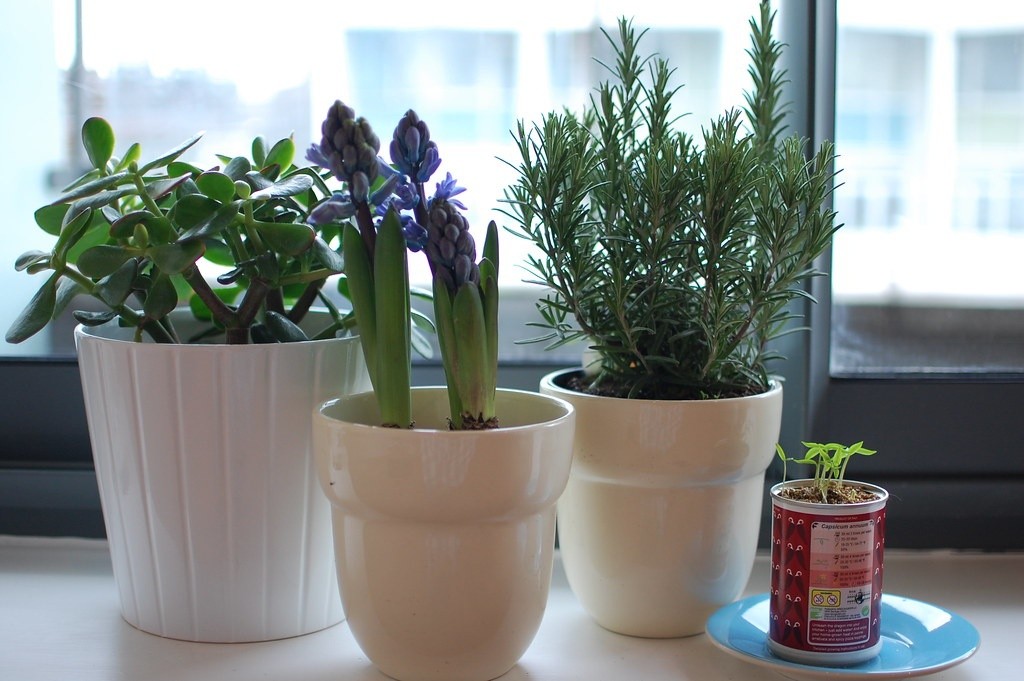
[766,437,888,667]
[6,117,367,643]
[491,3,851,638]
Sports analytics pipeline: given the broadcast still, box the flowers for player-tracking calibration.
[303,95,513,432]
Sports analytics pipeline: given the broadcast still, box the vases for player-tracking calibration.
[314,387,576,680]
